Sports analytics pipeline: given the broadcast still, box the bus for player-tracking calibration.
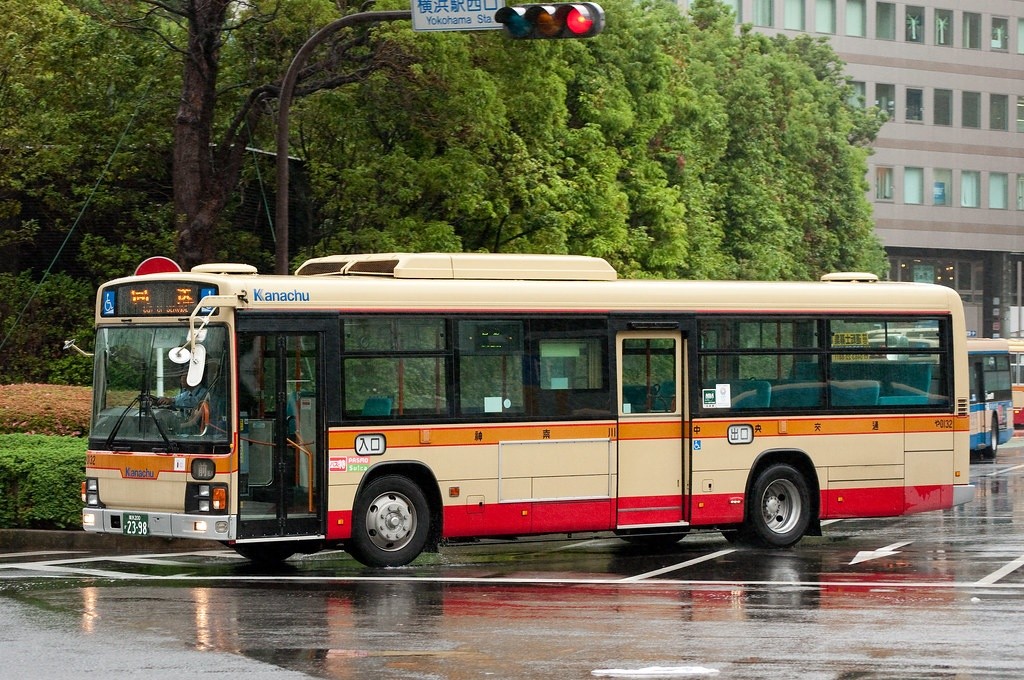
[1006,337,1024,427]
[63,250,974,568]
[865,327,1016,459]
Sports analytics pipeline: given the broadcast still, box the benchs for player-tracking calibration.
[790,361,931,398]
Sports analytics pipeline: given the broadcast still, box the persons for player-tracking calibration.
[158,372,214,422]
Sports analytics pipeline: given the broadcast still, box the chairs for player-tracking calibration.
[286,392,309,433]
[362,397,390,416]
[653,380,702,411]
[826,380,879,406]
[721,380,770,407]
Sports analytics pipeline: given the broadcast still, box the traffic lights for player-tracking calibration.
[494,1,604,39]
